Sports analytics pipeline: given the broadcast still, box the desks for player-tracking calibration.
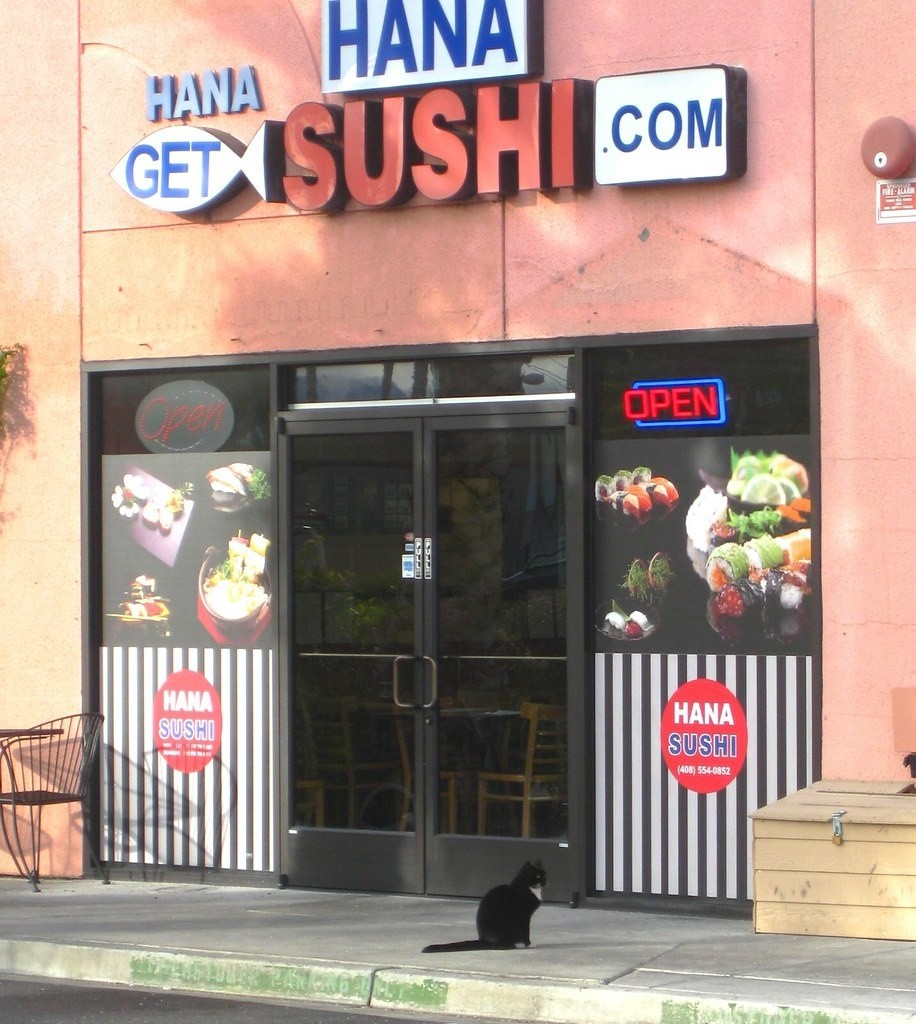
[360,700,522,835]
[0,727,63,741]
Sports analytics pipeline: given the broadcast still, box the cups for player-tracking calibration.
[199,545,273,637]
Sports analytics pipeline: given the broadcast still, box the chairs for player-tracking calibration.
[0,712,110,892]
[296,776,325,828]
[478,698,569,836]
[391,697,486,834]
[302,697,399,830]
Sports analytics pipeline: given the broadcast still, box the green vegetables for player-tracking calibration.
[248,468,273,501]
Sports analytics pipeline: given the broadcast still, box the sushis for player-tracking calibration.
[207,463,255,497]
[595,450,811,639]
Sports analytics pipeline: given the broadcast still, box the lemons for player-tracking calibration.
[778,478,802,504]
[741,473,786,506]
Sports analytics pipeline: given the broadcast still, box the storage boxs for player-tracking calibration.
[748,779,916,943]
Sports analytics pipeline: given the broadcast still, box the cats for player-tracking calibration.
[420,858,548,953]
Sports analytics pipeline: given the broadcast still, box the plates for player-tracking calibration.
[593,598,658,650]
